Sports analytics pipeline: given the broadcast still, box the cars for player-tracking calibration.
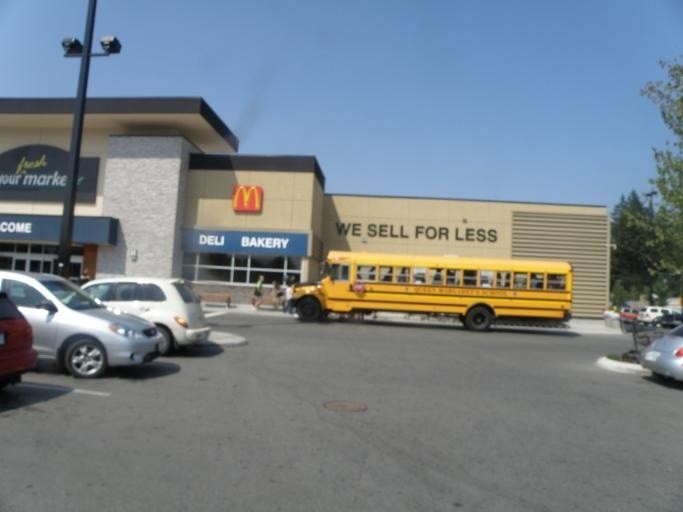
[0,270,210,389]
[620,305,683,382]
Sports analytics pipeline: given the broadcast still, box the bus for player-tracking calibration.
[289,250,574,332]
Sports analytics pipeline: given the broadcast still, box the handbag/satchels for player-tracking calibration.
[255,289,261,295]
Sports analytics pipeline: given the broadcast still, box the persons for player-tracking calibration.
[251,275,264,311]
[80,269,91,284]
[272,275,297,313]
